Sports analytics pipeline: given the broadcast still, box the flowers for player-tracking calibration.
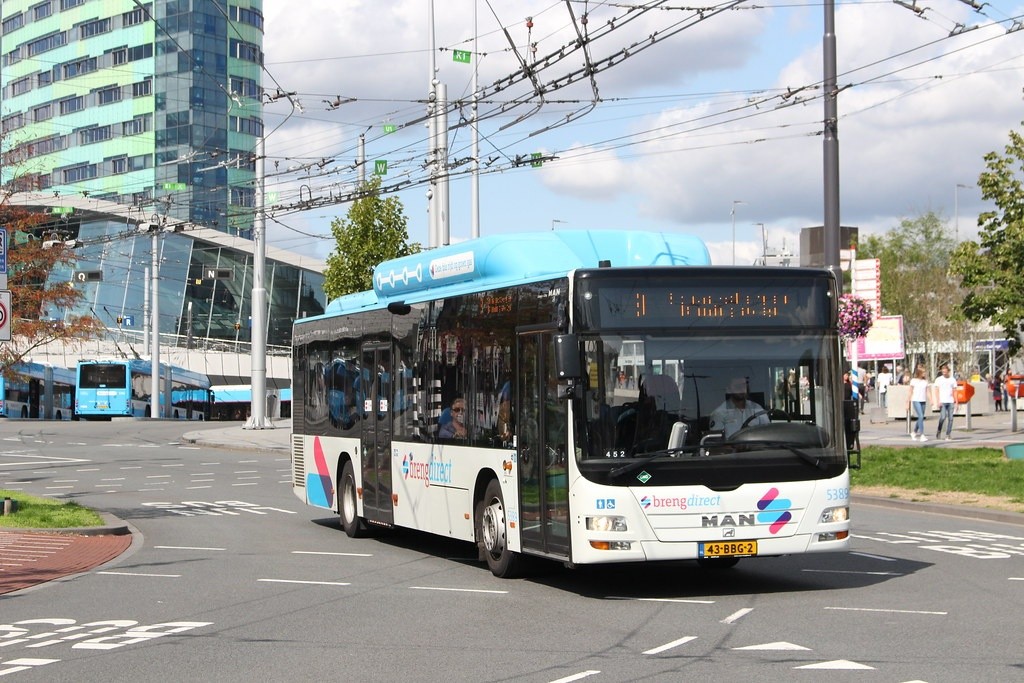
[837,293,873,342]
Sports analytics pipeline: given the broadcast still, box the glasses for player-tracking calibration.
[452,408,465,413]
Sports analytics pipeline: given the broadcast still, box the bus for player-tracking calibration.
[209,384,292,423]
[289,230,863,576]
[74,356,215,422]
[0,360,76,421]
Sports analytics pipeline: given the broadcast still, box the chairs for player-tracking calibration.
[320,361,681,459]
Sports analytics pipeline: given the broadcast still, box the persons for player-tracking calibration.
[934,364,958,442]
[778,355,910,415]
[906,364,934,442]
[439,398,466,439]
[709,369,771,440]
[993,368,1013,411]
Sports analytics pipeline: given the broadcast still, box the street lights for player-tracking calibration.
[732,200,745,265]
[754,222,767,266]
[953,182,973,247]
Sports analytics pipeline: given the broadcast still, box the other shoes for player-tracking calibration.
[920,437,928,442]
[936,433,941,440]
[946,435,952,440]
[911,432,918,441]
